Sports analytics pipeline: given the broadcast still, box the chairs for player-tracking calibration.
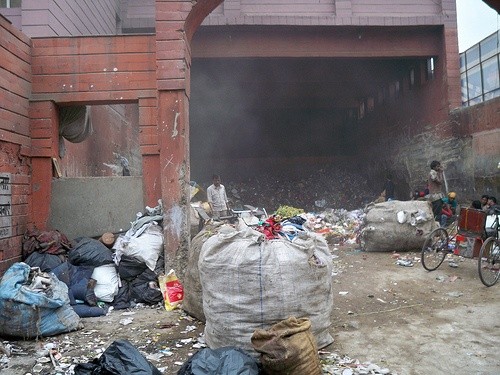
[458,206,495,239]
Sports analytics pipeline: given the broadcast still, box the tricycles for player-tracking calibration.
[421,207,500,287]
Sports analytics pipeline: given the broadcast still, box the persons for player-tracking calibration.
[207,174,230,217]
[428,161,445,226]
[418,192,500,228]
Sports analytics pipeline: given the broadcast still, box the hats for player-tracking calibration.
[448,191,455,198]
